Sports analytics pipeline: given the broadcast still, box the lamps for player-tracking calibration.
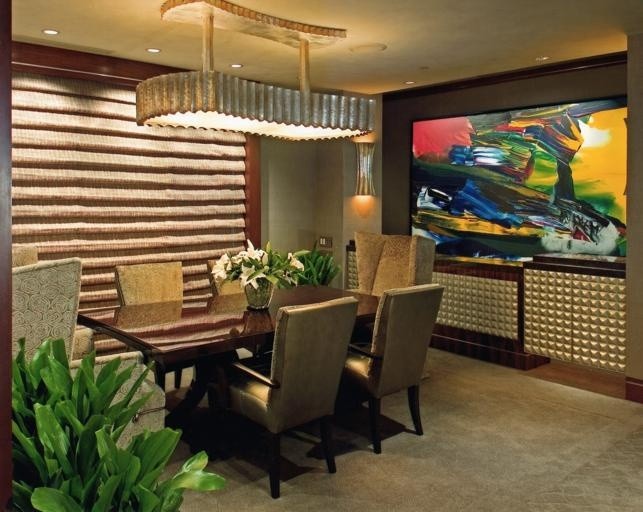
[127,0,378,146]
[346,127,380,219]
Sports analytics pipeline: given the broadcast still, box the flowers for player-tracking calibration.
[210,239,309,289]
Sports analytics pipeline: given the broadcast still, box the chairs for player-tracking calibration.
[339,229,437,382]
[341,284,446,456]
[8,242,167,462]
[107,260,184,304]
[206,255,246,297]
[201,295,361,500]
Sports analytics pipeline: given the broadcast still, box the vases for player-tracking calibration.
[241,275,275,312]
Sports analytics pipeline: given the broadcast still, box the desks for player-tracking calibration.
[73,282,381,467]
